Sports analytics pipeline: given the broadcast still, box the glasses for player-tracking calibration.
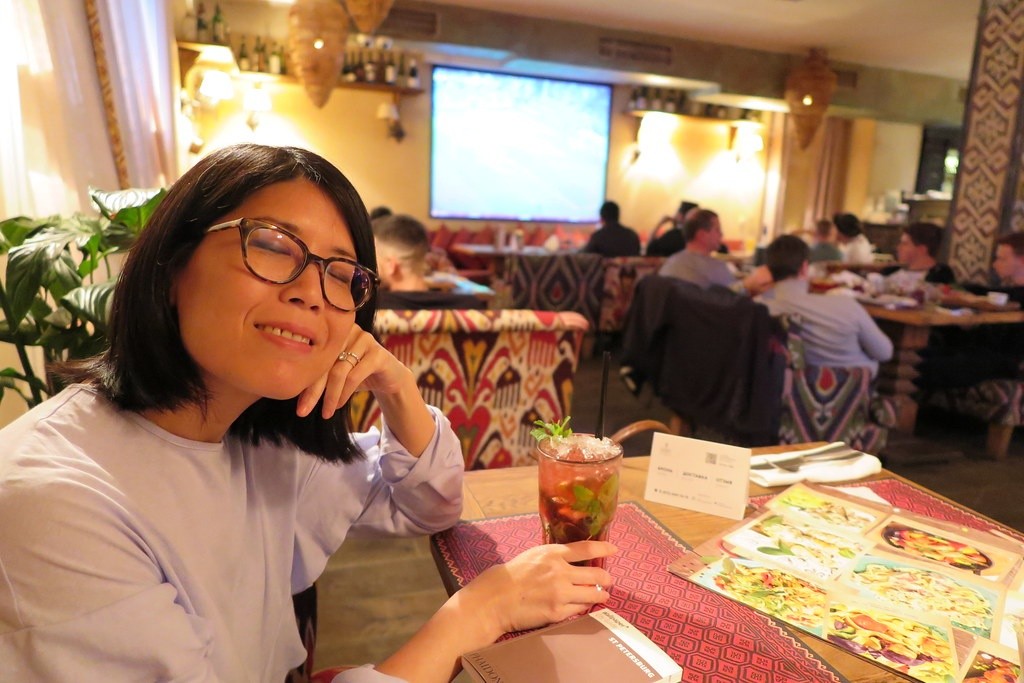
[205,217,381,312]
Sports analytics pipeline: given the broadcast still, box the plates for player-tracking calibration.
[707,504,995,681]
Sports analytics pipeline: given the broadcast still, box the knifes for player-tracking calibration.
[750,444,849,469]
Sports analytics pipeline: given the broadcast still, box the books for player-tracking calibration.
[461,608,683,683]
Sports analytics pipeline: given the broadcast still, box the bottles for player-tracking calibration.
[627,86,753,122]
[183,2,419,88]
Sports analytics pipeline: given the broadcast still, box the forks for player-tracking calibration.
[767,451,863,472]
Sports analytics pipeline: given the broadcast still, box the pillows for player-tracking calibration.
[432,223,589,250]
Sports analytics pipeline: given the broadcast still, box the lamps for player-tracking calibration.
[784,48,835,150]
[288,0,395,109]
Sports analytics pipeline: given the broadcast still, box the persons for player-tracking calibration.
[880,222,955,284]
[808,220,845,265]
[0,144,618,683]
[646,202,699,256]
[372,214,442,292]
[658,210,737,287]
[729,235,893,382]
[834,214,873,263]
[585,201,641,256]
[966,232,1024,309]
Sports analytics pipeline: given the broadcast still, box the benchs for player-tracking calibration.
[917,378,1024,459]
[351,250,897,471]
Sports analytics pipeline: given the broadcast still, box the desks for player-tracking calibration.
[429,442,1024,683]
[865,305,1024,435]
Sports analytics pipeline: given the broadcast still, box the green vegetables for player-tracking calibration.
[529,416,572,443]
[573,473,620,540]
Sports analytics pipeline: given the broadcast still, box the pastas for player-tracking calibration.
[720,569,824,629]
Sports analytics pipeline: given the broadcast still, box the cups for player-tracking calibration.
[536,434,624,569]
[865,272,884,297]
[987,292,1009,305]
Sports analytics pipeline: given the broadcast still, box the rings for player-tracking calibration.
[339,351,359,368]
